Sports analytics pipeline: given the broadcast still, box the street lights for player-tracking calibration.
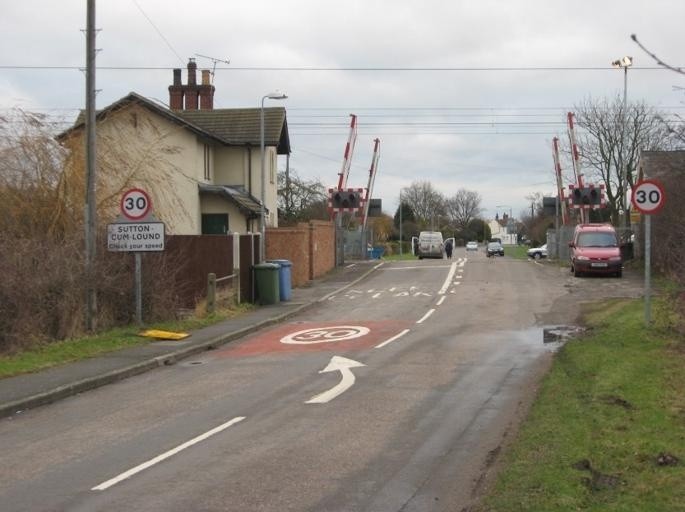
[497,206,512,245]
[258,93,288,265]
[612,54,634,241]
[400,187,409,256]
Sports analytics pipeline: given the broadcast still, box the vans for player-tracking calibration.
[567,221,625,279]
[411,230,456,261]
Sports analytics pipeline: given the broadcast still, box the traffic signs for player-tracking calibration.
[121,187,149,219]
[631,180,666,215]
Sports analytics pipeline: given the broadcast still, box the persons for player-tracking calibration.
[445,239,454,259]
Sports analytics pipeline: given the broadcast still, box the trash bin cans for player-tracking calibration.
[254,263,280,304]
[266,260,291,301]
[369,247,383,258]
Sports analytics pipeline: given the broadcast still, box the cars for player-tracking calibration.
[527,243,549,261]
[485,241,505,258]
[465,240,479,253]
